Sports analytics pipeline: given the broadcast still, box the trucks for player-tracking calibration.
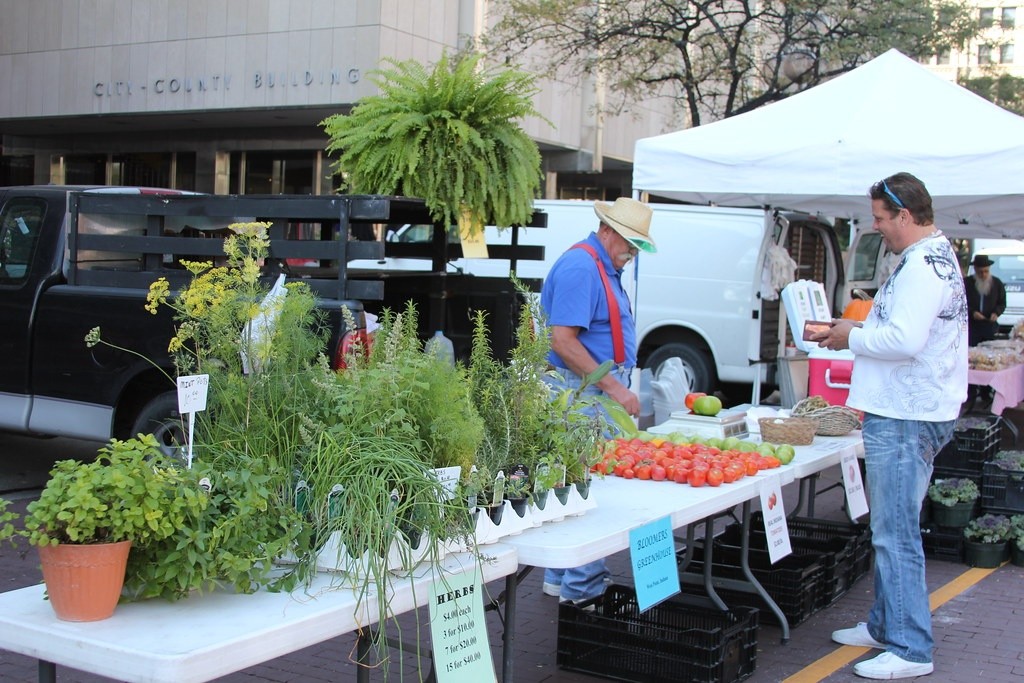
[347,195,889,401]
[971,236,1024,329]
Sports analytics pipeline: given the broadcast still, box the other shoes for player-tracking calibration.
[543,577,636,613]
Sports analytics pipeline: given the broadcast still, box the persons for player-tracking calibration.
[809,173,967,680]
[541,196,657,614]
[964,256,1008,408]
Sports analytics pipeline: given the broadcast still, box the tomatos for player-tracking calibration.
[685,392,722,416]
[588,431,796,487]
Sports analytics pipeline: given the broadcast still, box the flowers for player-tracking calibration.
[927,478,980,508]
[964,514,1011,543]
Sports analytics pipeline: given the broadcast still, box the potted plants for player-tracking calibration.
[1010,517,1024,565]
[0,232,638,623]
[982,450,1024,513]
[933,415,992,472]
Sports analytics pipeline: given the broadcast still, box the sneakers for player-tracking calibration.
[832,622,889,649]
[853,650,934,679]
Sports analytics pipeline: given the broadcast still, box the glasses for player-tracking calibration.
[877,179,903,207]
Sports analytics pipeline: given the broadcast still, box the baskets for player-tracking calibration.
[758,417,819,446]
[790,394,862,434]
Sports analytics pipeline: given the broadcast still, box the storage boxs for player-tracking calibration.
[555,416,1024,683]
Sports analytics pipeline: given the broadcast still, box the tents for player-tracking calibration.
[633,48,1024,326]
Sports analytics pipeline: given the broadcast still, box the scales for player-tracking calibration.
[647,409,750,442]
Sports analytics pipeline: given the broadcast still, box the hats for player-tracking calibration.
[594,196,657,254]
[968,254,995,267]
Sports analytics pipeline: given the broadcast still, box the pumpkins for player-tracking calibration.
[843,288,875,322]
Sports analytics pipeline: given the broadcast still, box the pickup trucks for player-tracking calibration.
[0,182,547,478]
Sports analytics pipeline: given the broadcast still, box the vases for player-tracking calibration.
[964,538,1007,569]
[932,500,974,528]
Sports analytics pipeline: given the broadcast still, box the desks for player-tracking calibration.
[1,403,865,683]
